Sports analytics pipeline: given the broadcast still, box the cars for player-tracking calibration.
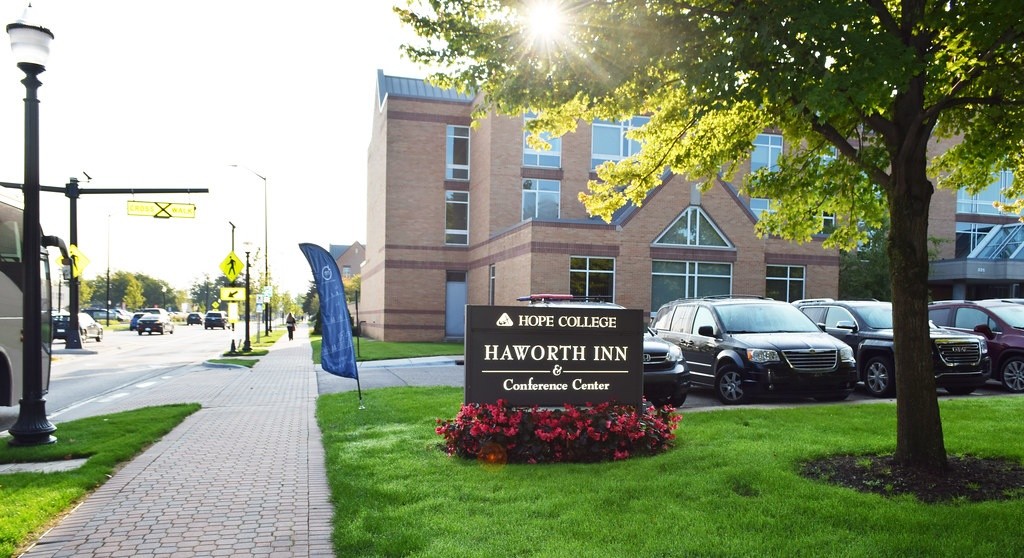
[129,307,174,335]
[82,309,132,321]
[186,312,205,325]
[52,312,103,343]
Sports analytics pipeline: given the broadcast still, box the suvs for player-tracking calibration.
[517,293,691,410]
[649,294,858,407]
[791,297,993,400]
[204,311,228,330]
[930,298,1024,394]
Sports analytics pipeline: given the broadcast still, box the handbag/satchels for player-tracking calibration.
[292,326,295,331]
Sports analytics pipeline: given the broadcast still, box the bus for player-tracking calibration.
[0,200,74,407]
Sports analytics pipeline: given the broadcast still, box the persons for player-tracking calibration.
[301,315,303,321]
[286,313,296,340]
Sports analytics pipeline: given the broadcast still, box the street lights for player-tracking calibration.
[228,163,269,337]
[7,2,58,448]
